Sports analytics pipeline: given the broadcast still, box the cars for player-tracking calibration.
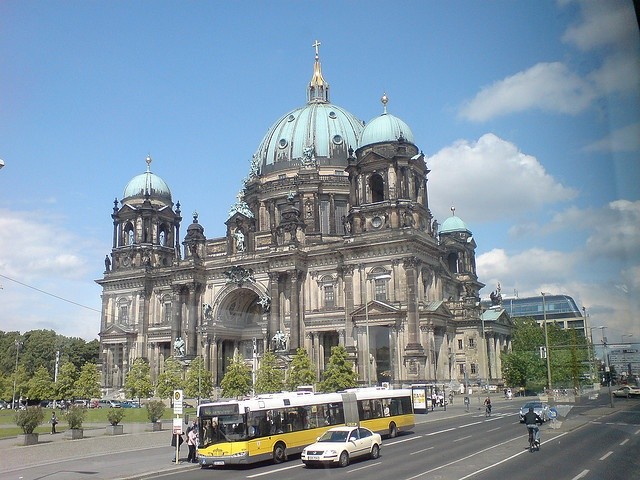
[173,401,193,409]
[514,389,536,396]
[519,401,551,423]
[49,402,63,408]
[301,426,383,468]
[612,385,640,398]
[120,401,138,408]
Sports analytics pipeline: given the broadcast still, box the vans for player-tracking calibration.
[100,400,121,408]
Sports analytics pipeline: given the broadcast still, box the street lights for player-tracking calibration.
[590,326,597,384]
[621,334,633,385]
[12,341,24,409]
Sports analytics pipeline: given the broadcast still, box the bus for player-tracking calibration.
[195,390,416,467]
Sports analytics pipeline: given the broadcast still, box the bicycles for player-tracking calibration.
[485,404,491,418]
[528,423,541,453]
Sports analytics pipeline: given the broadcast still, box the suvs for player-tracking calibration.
[0,400,6,410]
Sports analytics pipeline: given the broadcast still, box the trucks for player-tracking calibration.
[73,400,88,409]
[296,386,313,391]
[87,399,99,407]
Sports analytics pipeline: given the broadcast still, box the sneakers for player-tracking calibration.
[534,439,540,444]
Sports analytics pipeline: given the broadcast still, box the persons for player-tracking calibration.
[171,432,184,461]
[374,401,391,417]
[173,338,180,355]
[279,331,286,350]
[524,406,542,446]
[431,392,453,408]
[272,331,280,348]
[484,396,491,414]
[177,337,185,355]
[186,421,219,463]
[233,227,244,251]
[48,412,56,434]
[243,406,334,437]
